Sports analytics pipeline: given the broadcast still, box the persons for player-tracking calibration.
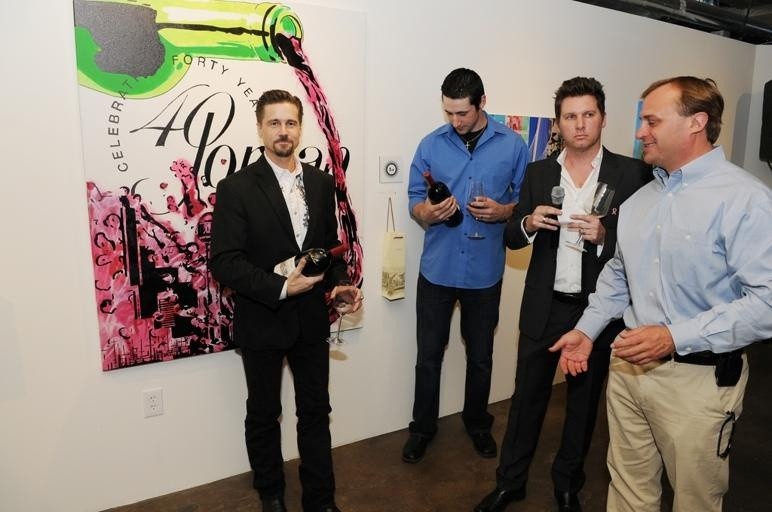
[548,74,772,511]
[209,88,364,511]
[402,67,530,464]
[471,75,655,511]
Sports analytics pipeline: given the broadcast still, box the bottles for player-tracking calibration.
[423,171,464,228]
[273,244,351,277]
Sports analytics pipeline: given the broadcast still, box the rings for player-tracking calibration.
[543,217,546,223]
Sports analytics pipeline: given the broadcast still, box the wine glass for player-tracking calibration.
[466,179,486,239]
[324,280,356,347]
[563,181,616,254]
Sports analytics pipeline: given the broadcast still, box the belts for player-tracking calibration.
[551,289,582,303]
[674,348,747,367]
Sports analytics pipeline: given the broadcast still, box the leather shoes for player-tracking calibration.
[462,420,498,459]
[552,480,584,512]
[400,421,439,465]
[261,497,285,511]
[472,484,528,512]
